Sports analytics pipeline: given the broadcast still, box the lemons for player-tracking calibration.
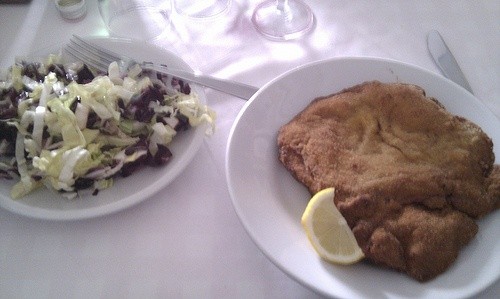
[301,186,366,265]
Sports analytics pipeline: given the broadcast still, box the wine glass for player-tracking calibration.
[252,0,313,41]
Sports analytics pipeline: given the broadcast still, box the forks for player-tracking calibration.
[64,34,260,101]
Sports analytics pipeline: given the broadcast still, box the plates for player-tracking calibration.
[224,56,500,299]
[0,35,207,221]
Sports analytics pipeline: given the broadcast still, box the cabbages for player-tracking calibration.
[1,51,217,199]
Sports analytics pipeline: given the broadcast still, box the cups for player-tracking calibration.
[98,0,171,36]
[174,0,230,18]
